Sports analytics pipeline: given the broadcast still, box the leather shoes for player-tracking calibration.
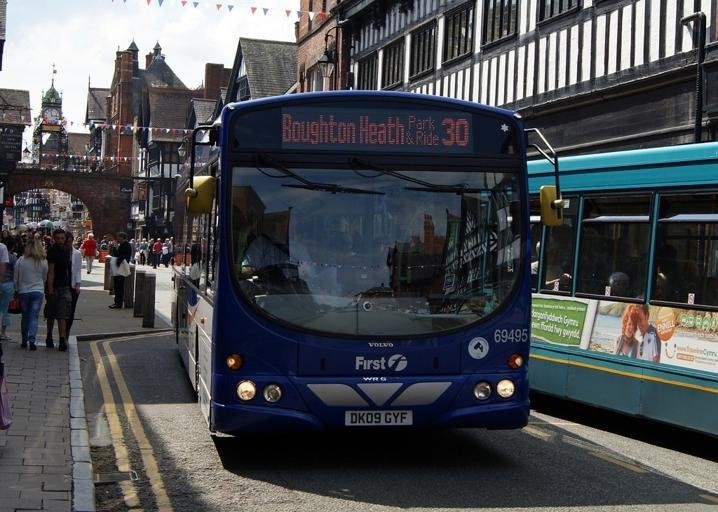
[108,303,123,309]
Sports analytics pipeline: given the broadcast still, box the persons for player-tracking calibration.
[80,233,97,274]
[531,241,701,304]
[0,224,173,268]
[44,229,70,351]
[636,304,661,362]
[14,239,49,350]
[109,232,132,309]
[65,232,82,341]
[241,211,319,289]
[0,243,9,295]
[615,304,637,358]
[0,236,17,340]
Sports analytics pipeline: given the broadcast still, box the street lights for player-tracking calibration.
[317,24,339,89]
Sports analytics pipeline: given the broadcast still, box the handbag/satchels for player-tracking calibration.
[0,364,14,430]
[7,299,22,314]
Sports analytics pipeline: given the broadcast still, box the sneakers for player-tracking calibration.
[45,336,69,351]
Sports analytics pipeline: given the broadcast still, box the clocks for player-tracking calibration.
[42,107,61,125]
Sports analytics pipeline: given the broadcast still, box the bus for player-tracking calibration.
[173,90,564,434]
[527,142,718,439]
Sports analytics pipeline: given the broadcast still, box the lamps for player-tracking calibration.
[176,137,191,157]
[315,25,340,78]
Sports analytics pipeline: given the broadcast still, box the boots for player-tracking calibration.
[29,342,37,350]
[21,339,27,348]
